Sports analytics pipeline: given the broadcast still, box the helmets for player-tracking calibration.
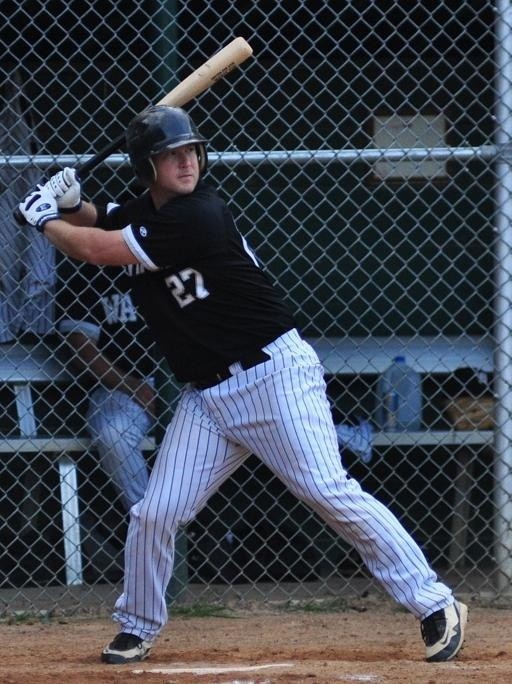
[125,104,209,186]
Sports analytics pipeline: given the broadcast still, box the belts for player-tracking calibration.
[192,348,270,390]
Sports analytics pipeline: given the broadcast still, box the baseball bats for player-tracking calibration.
[13,34,254,226]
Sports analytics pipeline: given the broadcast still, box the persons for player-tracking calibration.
[56,173,190,524]
[18,104,468,667]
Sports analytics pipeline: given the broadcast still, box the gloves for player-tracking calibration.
[42,167,81,215]
[17,184,62,233]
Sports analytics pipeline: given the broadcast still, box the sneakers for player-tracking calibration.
[420,597,469,662]
[101,632,151,663]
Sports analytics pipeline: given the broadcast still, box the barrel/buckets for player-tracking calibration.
[375,356,423,431]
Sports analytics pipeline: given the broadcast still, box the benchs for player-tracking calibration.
[6,431,495,587]
